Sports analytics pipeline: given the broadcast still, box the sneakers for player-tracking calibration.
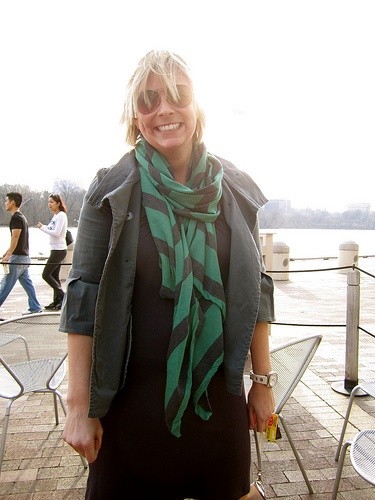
[22,310,42,316]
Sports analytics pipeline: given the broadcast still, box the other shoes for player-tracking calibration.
[55,295,66,308]
[44,302,63,311]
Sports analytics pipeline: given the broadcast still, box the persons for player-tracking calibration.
[57,49,276,500]
[0,192,41,313]
[37,194,67,310]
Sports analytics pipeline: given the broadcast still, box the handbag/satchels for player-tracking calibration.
[65,230,74,246]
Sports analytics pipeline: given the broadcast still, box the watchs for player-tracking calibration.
[249,370,277,387]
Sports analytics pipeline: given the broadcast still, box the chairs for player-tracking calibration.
[332,381,375,500]
[0,312,88,470]
[244,335,324,495]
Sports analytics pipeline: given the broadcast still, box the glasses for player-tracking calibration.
[136,84,192,115]
